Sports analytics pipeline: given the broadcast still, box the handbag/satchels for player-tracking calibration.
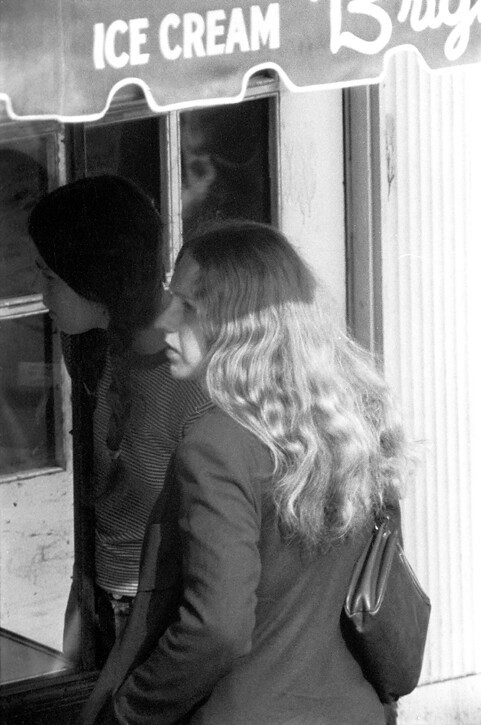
[342,514,431,705]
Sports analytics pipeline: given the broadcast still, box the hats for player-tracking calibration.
[28,175,161,303]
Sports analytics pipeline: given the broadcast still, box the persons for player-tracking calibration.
[28,174,215,672]
[78,220,422,725]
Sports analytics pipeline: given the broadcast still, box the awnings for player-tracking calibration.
[0,0,481,121]
